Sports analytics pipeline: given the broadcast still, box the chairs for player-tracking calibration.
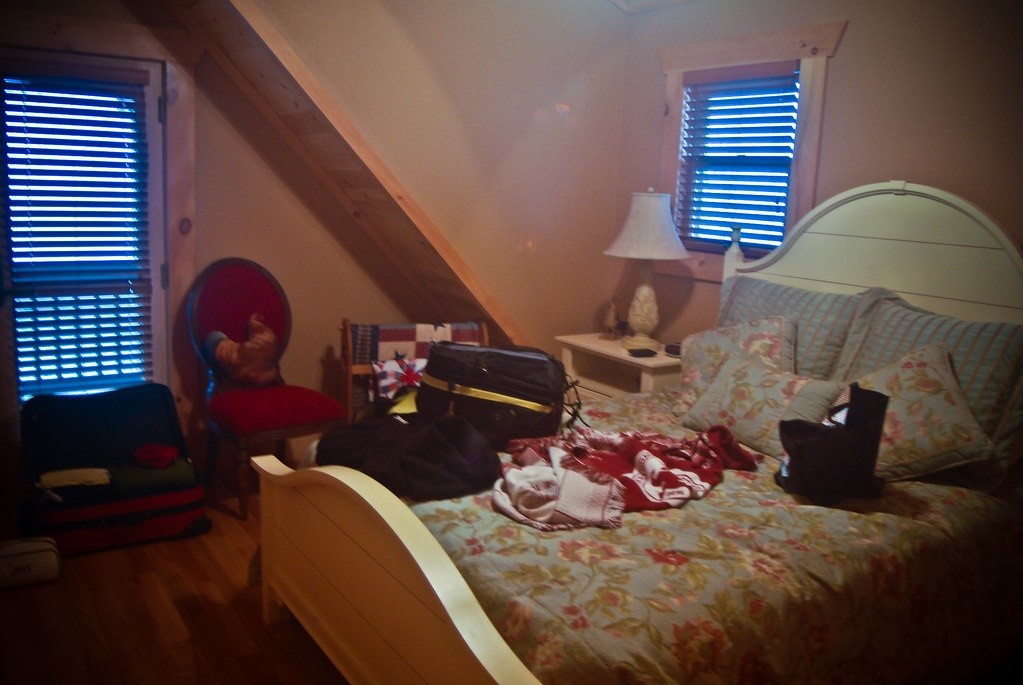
[186,249,349,523]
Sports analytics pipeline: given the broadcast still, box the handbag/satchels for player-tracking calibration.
[415,339,564,438]
[828,383,889,499]
[775,416,842,509]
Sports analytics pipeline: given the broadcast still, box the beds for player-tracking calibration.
[242,180,1023,685]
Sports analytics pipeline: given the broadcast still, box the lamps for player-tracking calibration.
[601,184,695,351]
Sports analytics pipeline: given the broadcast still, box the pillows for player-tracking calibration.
[832,286,1023,493]
[669,308,804,417]
[679,348,848,467]
[710,275,867,379]
[819,340,996,481]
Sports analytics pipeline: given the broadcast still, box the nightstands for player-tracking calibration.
[555,325,686,405]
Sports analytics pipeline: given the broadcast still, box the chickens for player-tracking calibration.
[208,313,280,388]
